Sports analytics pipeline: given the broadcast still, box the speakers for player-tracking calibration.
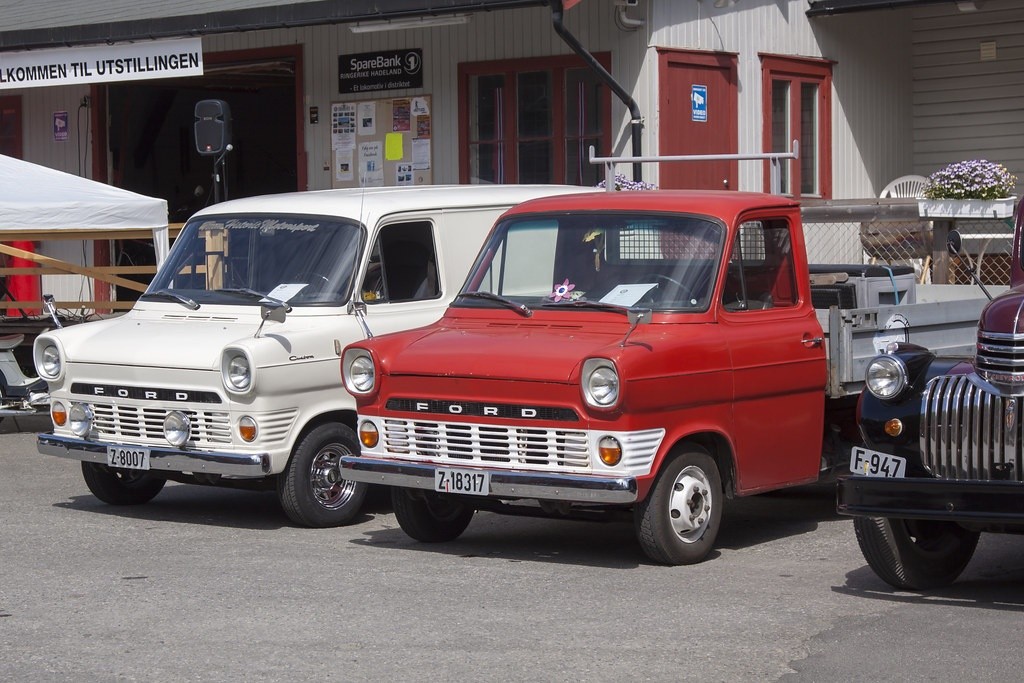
[194,99,233,155]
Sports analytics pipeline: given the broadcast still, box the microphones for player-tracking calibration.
[215,144,233,167]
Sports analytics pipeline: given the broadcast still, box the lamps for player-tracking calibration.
[348,14,475,33]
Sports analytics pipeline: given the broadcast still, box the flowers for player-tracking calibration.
[920,160,1016,200]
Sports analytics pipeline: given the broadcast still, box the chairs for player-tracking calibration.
[863,175,936,285]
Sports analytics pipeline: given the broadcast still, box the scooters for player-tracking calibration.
[0,294,64,408]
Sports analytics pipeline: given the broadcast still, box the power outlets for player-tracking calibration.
[79,95,89,110]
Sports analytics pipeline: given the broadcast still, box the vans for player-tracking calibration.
[33,183,607,527]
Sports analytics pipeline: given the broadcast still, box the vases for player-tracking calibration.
[917,197,1018,219]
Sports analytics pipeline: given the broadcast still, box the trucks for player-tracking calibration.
[339,189,1012,566]
[836,192,1024,591]
